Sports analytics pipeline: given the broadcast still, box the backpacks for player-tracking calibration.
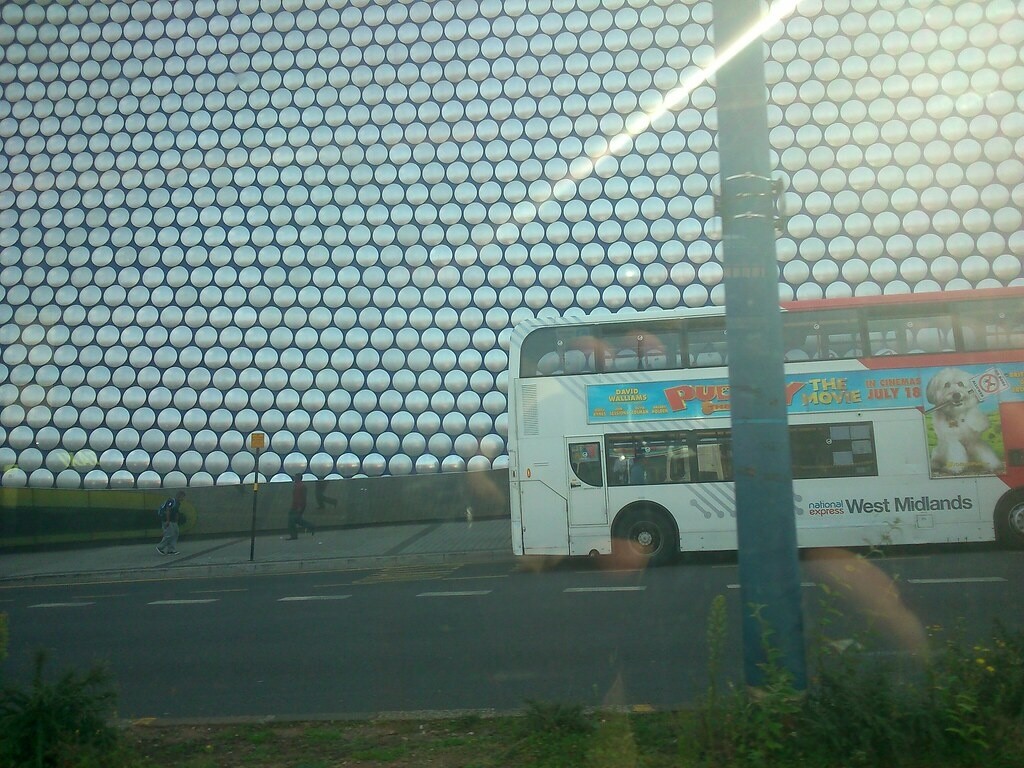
[158,497,176,518]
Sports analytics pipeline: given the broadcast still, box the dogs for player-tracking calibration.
[925,367,1001,474]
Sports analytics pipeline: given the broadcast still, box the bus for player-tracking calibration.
[507,285,1024,568]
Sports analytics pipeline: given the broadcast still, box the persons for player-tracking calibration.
[612,446,651,485]
[284,473,316,542]
[314,480,337,510]
[156,491,186,555]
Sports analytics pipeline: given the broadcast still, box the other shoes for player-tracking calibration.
[317,506,326,509]
[334,499,337,509]
[285,536,298,540]
[311,524,316,536]
[167,551,180,556]
[156,546,166,556]
